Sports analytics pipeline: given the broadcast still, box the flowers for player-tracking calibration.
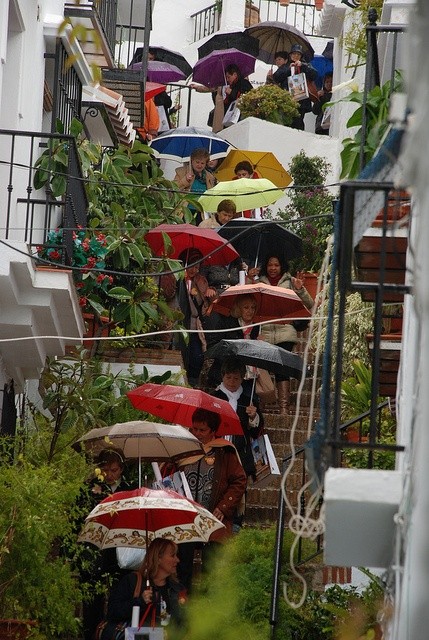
[35,223,114,308]
[308,282,375,376]
[260,185,335,272]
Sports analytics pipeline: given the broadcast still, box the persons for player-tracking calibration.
[134,98,161,178]
[174,148,215,228]
[141,49,156,62]
[207,293,260,393]
[266,51,290,84]
[312,71,333,135]
[197,199,236,281]
[223,64,254,129]
[272,44,319,130]
[209,360,265,531]
[190,84,225,133]
[163,247,217,387]
[232,160,263,218]
[205,159,219,186]
[153,408,248,604]
[76,446,137,586]
[244,253,314,415]
[147,76,182,136]
[106,537,183,639]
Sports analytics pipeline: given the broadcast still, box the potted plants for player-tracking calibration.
[286,148,334,217]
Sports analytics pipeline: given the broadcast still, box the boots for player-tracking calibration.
[277,380,290,415]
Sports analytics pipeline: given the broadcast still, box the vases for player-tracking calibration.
[79,312,116,347]
[290,270,326,316]
[340,420,361,444]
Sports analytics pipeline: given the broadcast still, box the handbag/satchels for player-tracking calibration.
[124,627,164,640]
[95,620,131,640]
[287,66,309,102]
[293,320,311,332]
[255,367,277,403]
[306,78,321,103]
[250,433,281,489]
[151,470,194,502]
[238,441,253,472]
[222,102,241,127]
[155,105,170,133]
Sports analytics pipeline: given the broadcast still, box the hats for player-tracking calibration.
[288,44,304,59]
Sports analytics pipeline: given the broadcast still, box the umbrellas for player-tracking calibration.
[126,382,245,438]
[192,47,257,89]
[212,281,312,325]
[126,60,187,82]
[145,81,167,102]
[198,339,308,406]
[244,20,315,69]
[308,55,333,90]
[144,223,240,272]
[322,40,334,63]
[214,217,311,281]
[131,46,193,80]
[70,421,207,489]
[212,150,294,204]
[196,177,284,213]
[77,486,226,600]
[197,31,260,58]
[148,126,234,173]
